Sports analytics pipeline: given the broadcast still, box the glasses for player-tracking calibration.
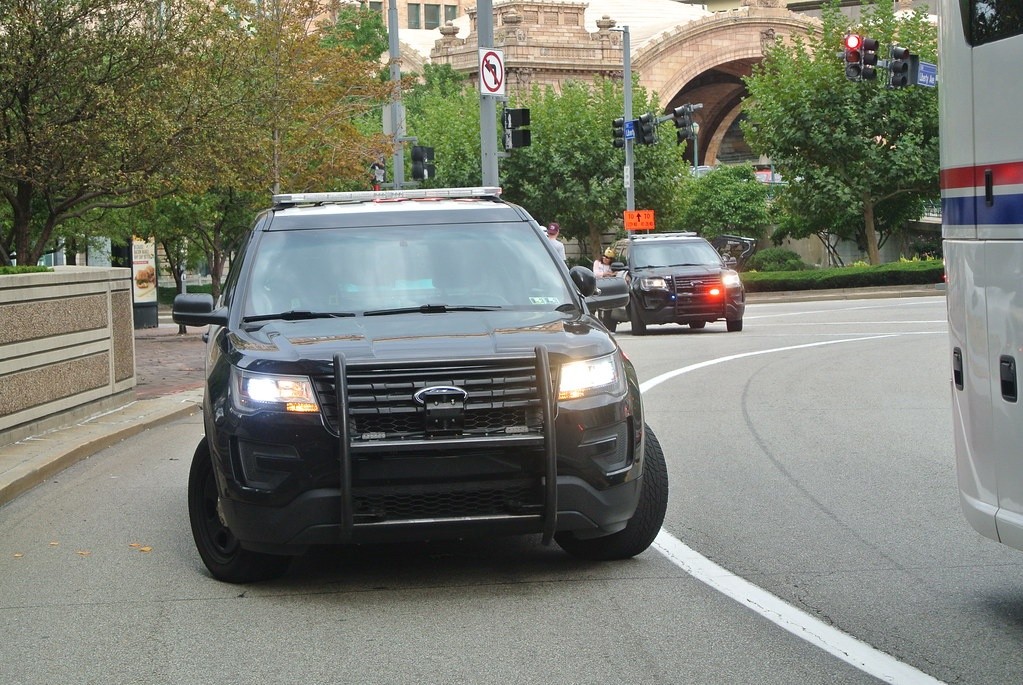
[602,254,611,259]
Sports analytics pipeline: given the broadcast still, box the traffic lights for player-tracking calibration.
[501,108,531,153]
[639,112,656,145]
[612,117,625,148]
[411,146,436,180]
[861,38,879,80]
[845,34,862,81]
[633,118,643,143]
[674,103,694,143]
[890,46,909,88]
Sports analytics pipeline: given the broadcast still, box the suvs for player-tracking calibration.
[598,232,746,336]
[171,186,670,583]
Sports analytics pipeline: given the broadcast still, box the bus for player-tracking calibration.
[935,0,1023,552]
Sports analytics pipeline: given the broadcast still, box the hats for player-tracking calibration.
[547,223,560,235]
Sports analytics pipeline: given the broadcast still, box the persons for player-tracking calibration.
[546,223,565,263]
[590,247,617,325]
